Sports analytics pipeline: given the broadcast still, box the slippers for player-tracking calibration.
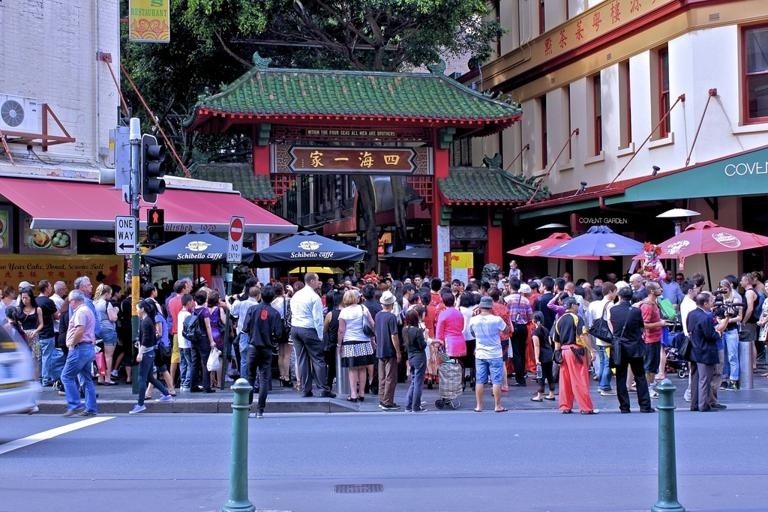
[474,407,482,412]
[494,407,507,412]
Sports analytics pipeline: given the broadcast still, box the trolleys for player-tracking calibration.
[433,337,461,410]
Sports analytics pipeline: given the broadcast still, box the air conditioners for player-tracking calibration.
[1,91,42,138]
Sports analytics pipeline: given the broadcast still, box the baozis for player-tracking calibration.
[53,232,69,246]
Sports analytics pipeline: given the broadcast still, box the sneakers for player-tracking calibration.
[594,374,665,399]
[161,394,172,402]
[129,404,146,414]
[582,408,599,414]
[621,409,631,413]
[180,386,216,393]
[640,408,656,413]
[720,381,728,390]
[255,411,262,418]
[729,381,739,389]
[378,401,428,414]
[683,390,727,412]
[64,409,97,417]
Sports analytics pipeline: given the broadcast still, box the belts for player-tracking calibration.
[75,341,92,347]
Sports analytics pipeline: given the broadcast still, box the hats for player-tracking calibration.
[479,295,495,309]
[18,281,35,288]
[380,290,397,304]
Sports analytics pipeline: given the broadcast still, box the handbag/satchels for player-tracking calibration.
[363,325,374,338]
[281,319,291,342]
[155,341,171,367]
[678,336,691,360]
[658,298,675,320]
[587,318,614,342]
[612,337,621,365]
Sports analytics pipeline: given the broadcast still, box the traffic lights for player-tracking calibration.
[139,132,167,205]
[146,206,167,243]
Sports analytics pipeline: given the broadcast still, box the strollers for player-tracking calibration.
[662,332,693,379]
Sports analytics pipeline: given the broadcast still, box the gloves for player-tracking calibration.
[554,349,563,364]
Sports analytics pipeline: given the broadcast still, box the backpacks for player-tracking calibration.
[182,307,203,341]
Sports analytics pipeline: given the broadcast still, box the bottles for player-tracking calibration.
[536,365,543,378]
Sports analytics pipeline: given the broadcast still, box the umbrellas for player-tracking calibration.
[507,232,574,278]
[541,226,644,278]
[140,232,255,286]
[249,230,367,281]
[381,246,432,259]
[632,220,768,291]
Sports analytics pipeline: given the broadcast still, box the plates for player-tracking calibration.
[31,235,51,248]
[0,218,7,249]
[51,232,70,247]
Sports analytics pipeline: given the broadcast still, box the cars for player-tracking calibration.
[1,312,43,421]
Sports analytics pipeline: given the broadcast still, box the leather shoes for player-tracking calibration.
[347,396,357,401]
[530,397,542,401]
[302,392,314,396]
[511,383,526,386]
[97,380,119,385]
[320,391,336,398]
[358,396,365,402]
[544,396,555,400]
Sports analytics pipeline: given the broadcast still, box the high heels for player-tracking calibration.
[279,376,294,388]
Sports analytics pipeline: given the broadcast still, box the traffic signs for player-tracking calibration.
[113,216,137,256]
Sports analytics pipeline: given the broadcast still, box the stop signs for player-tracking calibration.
[229,216,244,244]
